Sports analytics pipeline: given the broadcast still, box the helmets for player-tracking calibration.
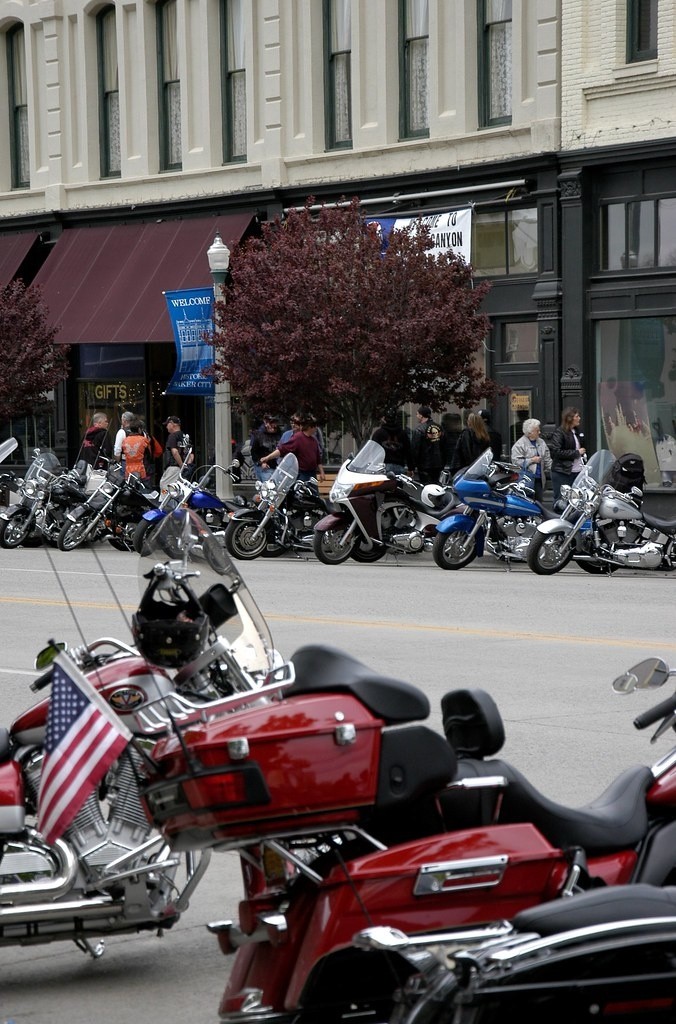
[131,580,209,668]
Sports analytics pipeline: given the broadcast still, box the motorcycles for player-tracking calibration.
[32,499,676,1023]
[132,434,290,559]
[433,446,621,574]
[351,881,676,1023]
[525,449,676,576]
[57,445,160,552]
[0,506,286,960]
[0,447,108,550]
[1,437,47,548]
[224,451,389,563]
[312,439,468,565]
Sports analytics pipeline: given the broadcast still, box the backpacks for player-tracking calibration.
[602,453,647,509]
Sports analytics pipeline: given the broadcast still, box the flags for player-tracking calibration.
[36,652,132,845]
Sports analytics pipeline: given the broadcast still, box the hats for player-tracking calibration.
[417,406,431,418]
[162,416,181,425]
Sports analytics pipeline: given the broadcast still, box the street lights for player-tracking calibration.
[205,233,232,503]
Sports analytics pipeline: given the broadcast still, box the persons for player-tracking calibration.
[511,418,552,504]
[451,413,490,477]
[655,426,676,487]
[550,407,586,500]
[441,413,463,467]
[250,410,325,490]
[402,407,444,482]
[82,412,163,486]
[372,414,414,471]
[478,410,502,462]
[161,415,194,481]
